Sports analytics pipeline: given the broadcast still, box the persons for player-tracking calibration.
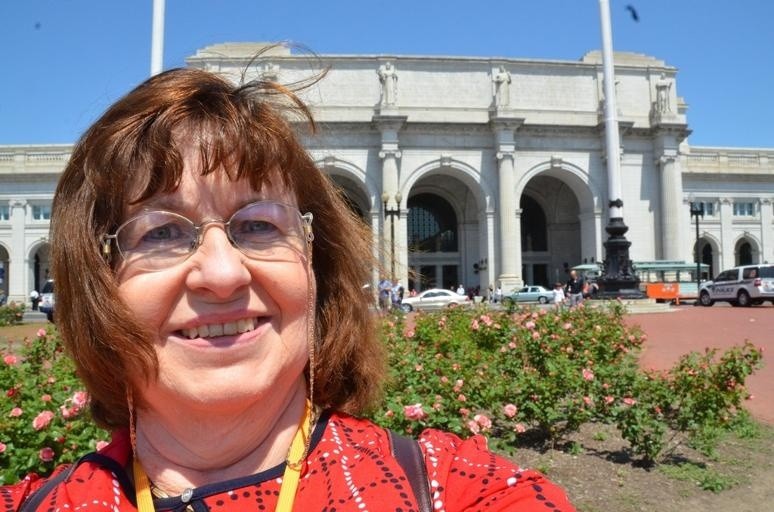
[488,284,501,304]
[31,288,39,310]
[411,288,416,297]
[378,273,404,309]
[0,69,578,512]
[450,283,464,295]
[553,269,591,311]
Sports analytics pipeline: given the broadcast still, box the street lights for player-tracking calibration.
[689,193,705,291]
[382,191,402,275]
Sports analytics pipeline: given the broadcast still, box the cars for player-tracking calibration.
[39,279,55,323]
[503,286,554,306]
[401,288,471,314]
[699,264,774,305]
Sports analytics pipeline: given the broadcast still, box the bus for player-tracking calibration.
[570,261,711,300]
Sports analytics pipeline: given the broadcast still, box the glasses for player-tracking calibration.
[100,196,314,273]
